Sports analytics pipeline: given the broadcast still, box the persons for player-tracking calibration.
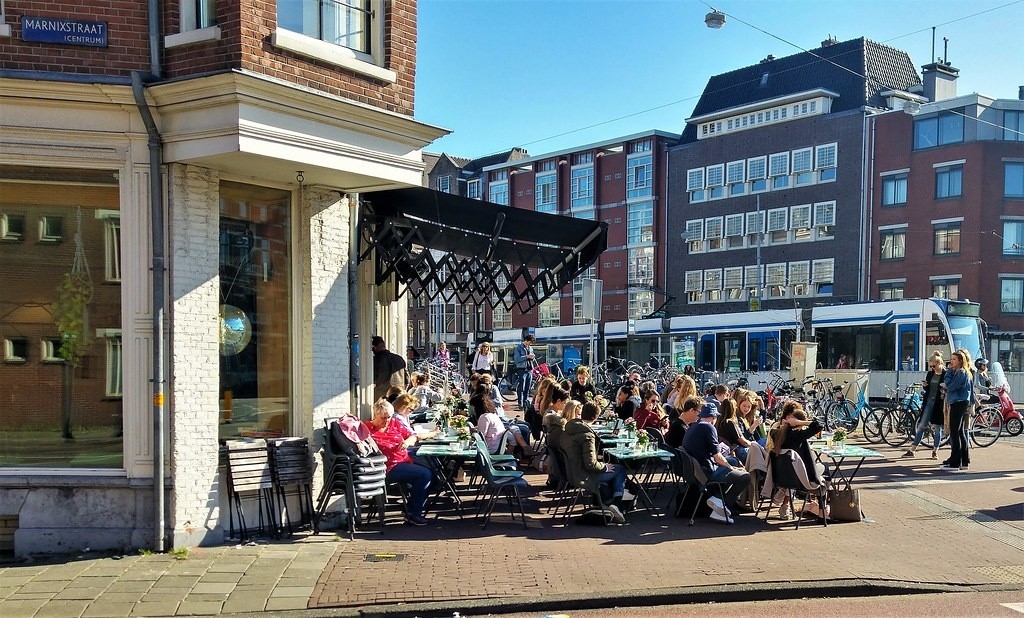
[684,403,751,525]
[974,358,1002,421]
[770,409,839,524]
[902,348,975,470]
[661,374,697,409]
[705,384,766,464]
[634,389,670,430]
[466,342,539,457]
[533,367,596,449]
[614,387,636,421]
[666,396,703,449]
[514,334,535,411]
[615,373,655,406]
[561,403,626,524]
[838,354,847,369]
[992,362,1011,396]
[436,342,449,367]
[364,336,444,525]
[765,401,803,520]
[453,393,506,482]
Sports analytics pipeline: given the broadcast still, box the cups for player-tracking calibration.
[449,430,455,437]
[621,430,628,439]
[634,447,642,456]
[817,431,822,439]
[617,442,625,450]
[450,443,460,449]
[609,422,614,429]
[827,438,832,449]
[827,433,833,440]
[461,440,469,449]
[437,433,445,438]
[836,441,844,449]
[835,447,842,452]
[639,443,647,452]
[617,419,623,427]
[629,443,636,451]
[630,432,635,439]
[649,442,658,452]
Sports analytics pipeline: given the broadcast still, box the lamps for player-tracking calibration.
[903,99,921,114]
[704,10,726,29]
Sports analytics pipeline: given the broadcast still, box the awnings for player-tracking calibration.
[357,186,607,315]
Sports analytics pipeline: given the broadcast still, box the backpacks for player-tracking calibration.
[335,413,379,458]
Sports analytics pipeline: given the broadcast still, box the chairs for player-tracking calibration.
[225,399,832,543]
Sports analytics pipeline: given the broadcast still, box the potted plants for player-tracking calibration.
[456,401,468,416]
[832,430,847,449]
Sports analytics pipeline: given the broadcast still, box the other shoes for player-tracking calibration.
[932,451,938,460]
[941,464,960,471]
[523,446,539,456]
[779,503,790,520]
[609,505,625,522]
[902,450,915,457]
[960,465,969,470]
[942,460,950,464]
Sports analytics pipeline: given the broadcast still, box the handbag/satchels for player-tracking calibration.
[828,477,861,521]
[531,446,552,473]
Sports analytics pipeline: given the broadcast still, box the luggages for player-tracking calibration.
[510,418,530,466]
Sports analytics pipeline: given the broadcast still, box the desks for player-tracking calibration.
[416,410,884,521]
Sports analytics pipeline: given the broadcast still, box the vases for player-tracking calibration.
[458,439,469,449]
[457,427,469,433]
[627,431,636,439]
[607,421,614,428]
[636,442,646,452]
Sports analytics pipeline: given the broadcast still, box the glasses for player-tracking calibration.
[632,377,641,382]
[648,398,659,403]
[482,345,489,347]
[930,362,938,368]
[407,407,414,411]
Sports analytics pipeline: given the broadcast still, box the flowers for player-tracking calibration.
[585,391,650,442]
[444,397,472,440]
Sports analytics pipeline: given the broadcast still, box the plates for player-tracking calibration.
[834,452,843,455]
[433,438,446,440]
[447,449,463,452]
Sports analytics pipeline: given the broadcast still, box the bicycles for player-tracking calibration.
[498,353,1024,448]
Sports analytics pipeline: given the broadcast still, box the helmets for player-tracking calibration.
[975,358,985,369]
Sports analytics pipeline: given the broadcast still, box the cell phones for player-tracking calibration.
[922,381,928,384]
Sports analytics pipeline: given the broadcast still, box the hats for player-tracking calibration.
[698,403,721,417]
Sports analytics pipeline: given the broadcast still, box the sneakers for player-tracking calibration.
[706,496,731,516]
[404,513,428,525]
[710,510,734,523]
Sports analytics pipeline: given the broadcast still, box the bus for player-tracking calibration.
[465,277,989,408]
